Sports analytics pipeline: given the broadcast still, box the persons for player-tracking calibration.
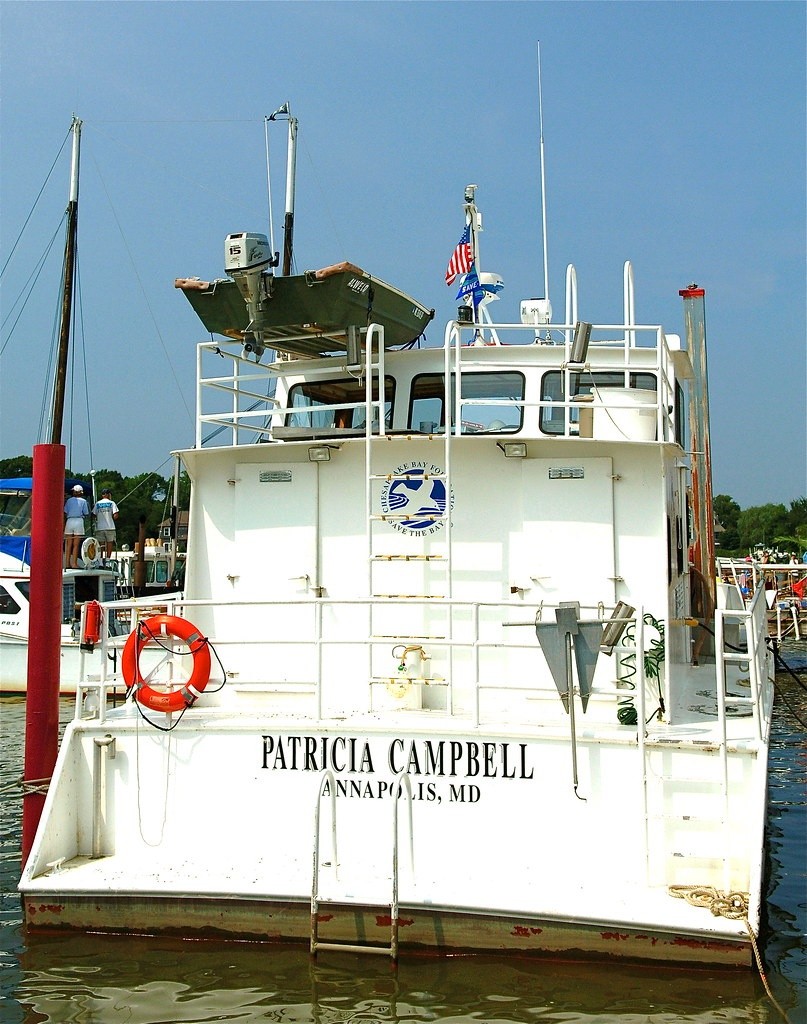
[64,485,89,568]
[745,549,798,564]
[92,489,119,558]
[789,600,798,611]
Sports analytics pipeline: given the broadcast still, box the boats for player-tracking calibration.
[1,40,782,974]
[174,101,436,359]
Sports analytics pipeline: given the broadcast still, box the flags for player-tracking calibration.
[791,577,807,599]
[445,222,473,286]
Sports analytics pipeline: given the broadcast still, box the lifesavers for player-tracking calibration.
[123,616,212,714]
[81,538,102,568]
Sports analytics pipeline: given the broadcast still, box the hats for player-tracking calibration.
[71,485,83,492]
[101,489,113,495]
